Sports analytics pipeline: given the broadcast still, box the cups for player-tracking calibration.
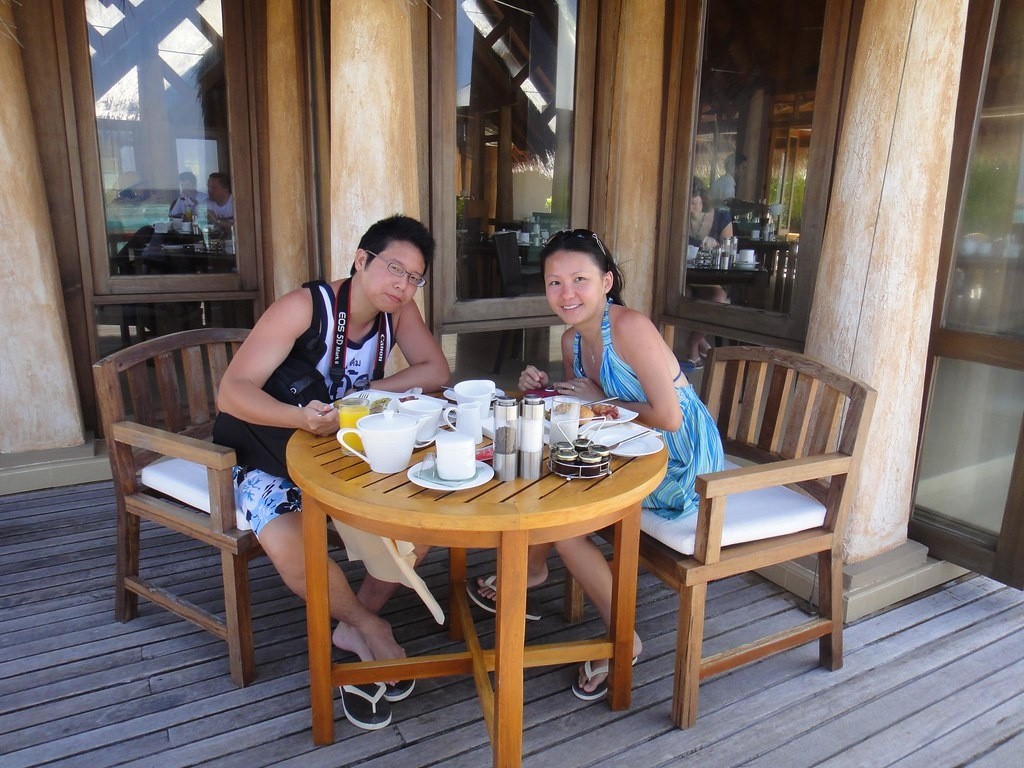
[553,439,609,476]
[172,218,182,229]
[182,222,191,230]
[751,230,760,238]
[454,380,493,416]
[435,434,476,480]
[520,233,529,244]
[400,401,443,442]
[531,217,535,222]
[339,399,371,456]
[549,395,580,451]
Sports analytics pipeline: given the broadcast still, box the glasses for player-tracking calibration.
[545,229,609,273]
[365,250,427,287]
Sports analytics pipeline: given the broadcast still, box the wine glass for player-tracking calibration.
[732,215,741,223]
[746,212,753,223]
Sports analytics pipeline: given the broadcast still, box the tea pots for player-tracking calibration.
[336,409,432,473]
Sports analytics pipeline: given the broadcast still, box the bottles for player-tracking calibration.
[697,251,712,269]
[480,232,485,242]
[520,394,545,480]
[764,223,774,241]
[738,249,755,269]
[767,209,770,219]
[728,237,738,267]
[721,237,730,269]
[713,246,722,266]
[534,234,539,247]
[493,398,520,481]
[443,403,483,444]
[186,205,192,222]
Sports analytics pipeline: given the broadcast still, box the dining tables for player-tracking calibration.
[283,385,671,768]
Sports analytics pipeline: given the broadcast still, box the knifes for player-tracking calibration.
[608,431,652,450]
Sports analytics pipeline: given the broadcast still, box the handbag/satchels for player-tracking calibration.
[212,279,332,482]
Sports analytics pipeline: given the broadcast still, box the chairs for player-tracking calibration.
[493,235,548,373]
[89,326,343,692]
[563,340,883,730]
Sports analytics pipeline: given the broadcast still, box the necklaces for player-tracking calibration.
[688,209,707,239]
[580,320,603,364]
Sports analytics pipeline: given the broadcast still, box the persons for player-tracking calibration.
[467,228,724,701]
[710,153,747,212]
[685,176,733,369]
[213,215,451,686]
[171,173,235,234]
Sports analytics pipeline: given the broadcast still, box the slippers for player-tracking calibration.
[335,658,392,730]
[466,575,542,620]
[382,678,416,701]
[571,656,638,701]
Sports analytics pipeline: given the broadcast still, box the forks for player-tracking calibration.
[318,393,369,416]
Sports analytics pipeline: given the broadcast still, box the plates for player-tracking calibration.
[542,396,639,435]
[587,427,664,457]
[175,230,192,234]
[750,239,760,241]
[407,459,494,491]
[443,386,505,401]
[329,388,450,410]
[517,244,530,246]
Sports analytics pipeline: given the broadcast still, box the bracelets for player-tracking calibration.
[216,217,221,224]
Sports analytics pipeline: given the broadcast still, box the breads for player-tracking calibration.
[556,403,593,424]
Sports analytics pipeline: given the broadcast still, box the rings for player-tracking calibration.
[572,385,575,391]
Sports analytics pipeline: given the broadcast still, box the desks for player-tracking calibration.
[466,240,543,300]
[140,231,235,339]
[687,267,772,405]
